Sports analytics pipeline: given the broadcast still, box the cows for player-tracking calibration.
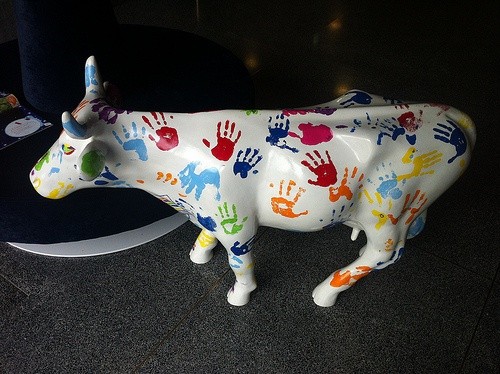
[29,55,477,307]
